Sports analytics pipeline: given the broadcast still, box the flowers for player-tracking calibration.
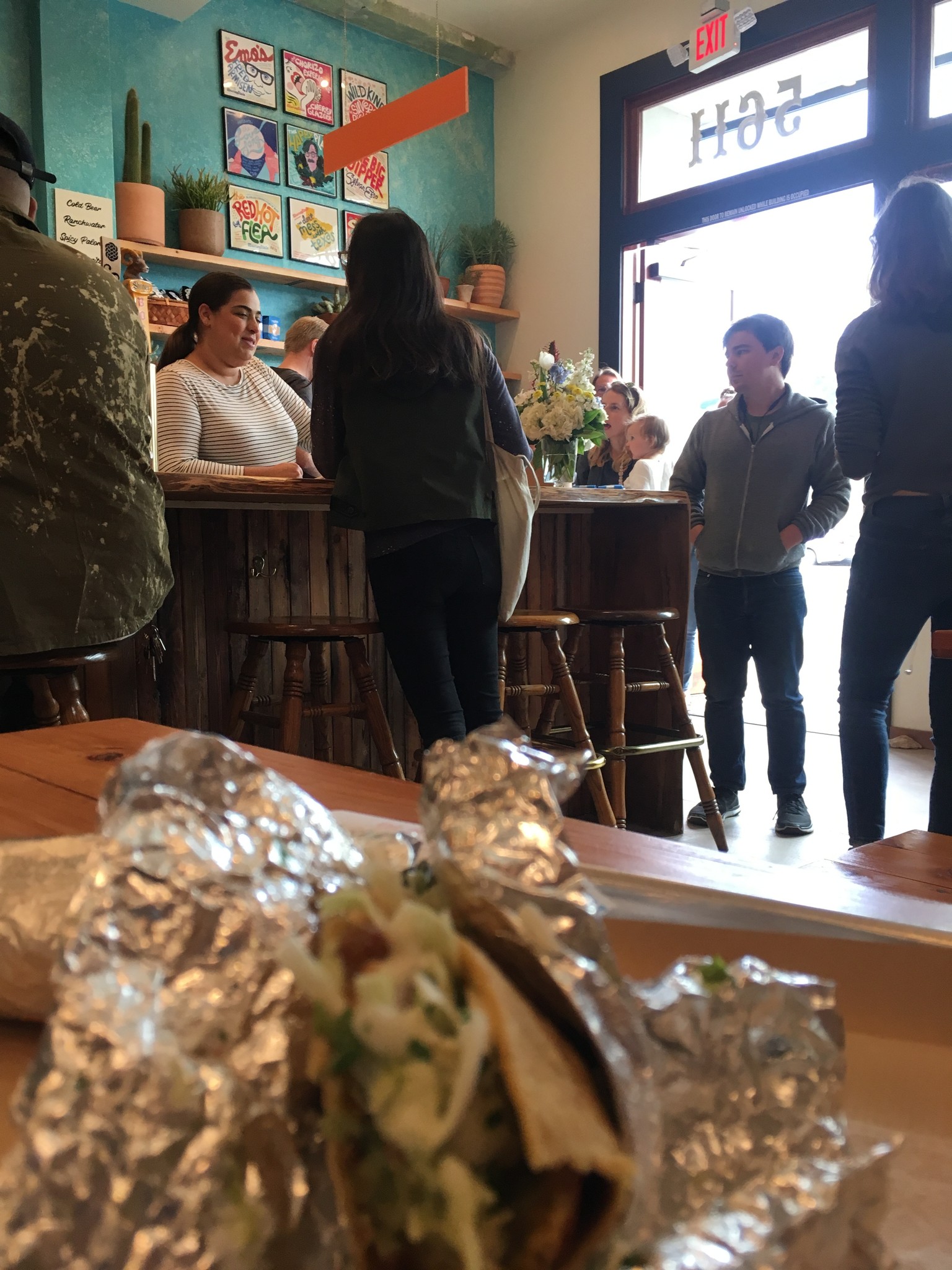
[512,339,607,442]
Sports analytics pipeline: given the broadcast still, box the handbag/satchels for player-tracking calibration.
[462,320,542,624]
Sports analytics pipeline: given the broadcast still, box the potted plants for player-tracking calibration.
[455,266,482,303]
[161,165,237,257]
[459,217,519,308]
[311,287,349,325]
[113,88,166,246]
[425,219,456,299]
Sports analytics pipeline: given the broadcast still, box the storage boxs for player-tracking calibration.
[147,296,191,327]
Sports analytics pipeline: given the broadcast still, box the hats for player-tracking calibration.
[0,111,57,191]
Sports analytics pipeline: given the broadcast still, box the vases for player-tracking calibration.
[338,68,388,127]
[284,123,338,198]
[221,106,281,185]
[540,436,578,487]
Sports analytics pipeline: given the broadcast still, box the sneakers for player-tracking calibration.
[687,787,742,827]
[775,794,814,834]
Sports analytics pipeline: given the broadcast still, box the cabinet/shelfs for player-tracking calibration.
[120,241,523,380]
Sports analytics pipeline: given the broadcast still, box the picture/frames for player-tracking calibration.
[342,209,367,260]
[218,28,278,110]
[341,150,390,211]
[226,181,284,258]
[280,48,335,127]
[287,197,340,269]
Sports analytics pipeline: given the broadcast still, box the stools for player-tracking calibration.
[491,611,625,832]
[219,614,410,781]
[588,604,734,853]
[0,648,127,729]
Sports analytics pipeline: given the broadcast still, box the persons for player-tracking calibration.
[623,413,675,493]
[591,367,625,406]
[310,209,536,795]
[684,384,741,687]
[154,270,315,483]
[669,312,850,837]
[0,112,172,733]
[267,316,331,478]
[831,175,952,851]
[572,379,648,488]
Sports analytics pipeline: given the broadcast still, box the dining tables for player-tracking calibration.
[0,720,952,1267]
